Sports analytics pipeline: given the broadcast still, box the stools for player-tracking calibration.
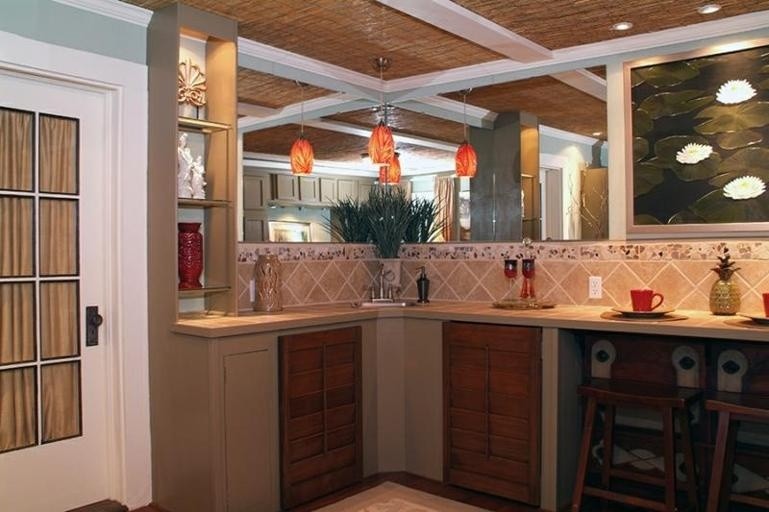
[571,377,704,512]
[705,388,769,512]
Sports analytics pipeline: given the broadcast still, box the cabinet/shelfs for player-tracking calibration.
[276,325,364,512]
[243,164,413,212]
[442,320,543,512]
[146,0,240,322]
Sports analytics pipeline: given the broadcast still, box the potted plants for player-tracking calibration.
[361,183,421,298]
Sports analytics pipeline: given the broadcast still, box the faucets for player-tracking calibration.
[377,264,385,298]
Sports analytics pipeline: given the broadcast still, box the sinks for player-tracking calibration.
[352,299,416,308]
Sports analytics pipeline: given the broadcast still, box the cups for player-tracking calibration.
[762,293,769,317]
[630,289,664,311]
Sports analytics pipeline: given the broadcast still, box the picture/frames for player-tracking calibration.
[622,35,769,241]
[265,220,311,244]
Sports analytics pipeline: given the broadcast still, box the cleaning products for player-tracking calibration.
[415,265,430,304]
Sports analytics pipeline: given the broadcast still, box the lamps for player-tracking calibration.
[454,90,477,178]
[291,82,314,176]
[379,149,401,186]
[368,58,395,164]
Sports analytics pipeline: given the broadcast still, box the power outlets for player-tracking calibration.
[588,276,603,300]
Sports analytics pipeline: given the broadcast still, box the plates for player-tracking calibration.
[736,313,769,323]
[611,308,675,317]
[493,301,556,309]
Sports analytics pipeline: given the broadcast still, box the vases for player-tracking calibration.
[253,254,284,312]
[179,222,204,290]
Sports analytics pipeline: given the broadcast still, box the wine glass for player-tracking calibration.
[520,258,535,297]
[504,259,517,300]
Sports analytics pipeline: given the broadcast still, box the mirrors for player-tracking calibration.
[236,50,384,244]
[384,57,611,244]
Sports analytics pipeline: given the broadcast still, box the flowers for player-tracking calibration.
[715,77,757,104]
[724,177,764,200]
[675,142,713,165]
